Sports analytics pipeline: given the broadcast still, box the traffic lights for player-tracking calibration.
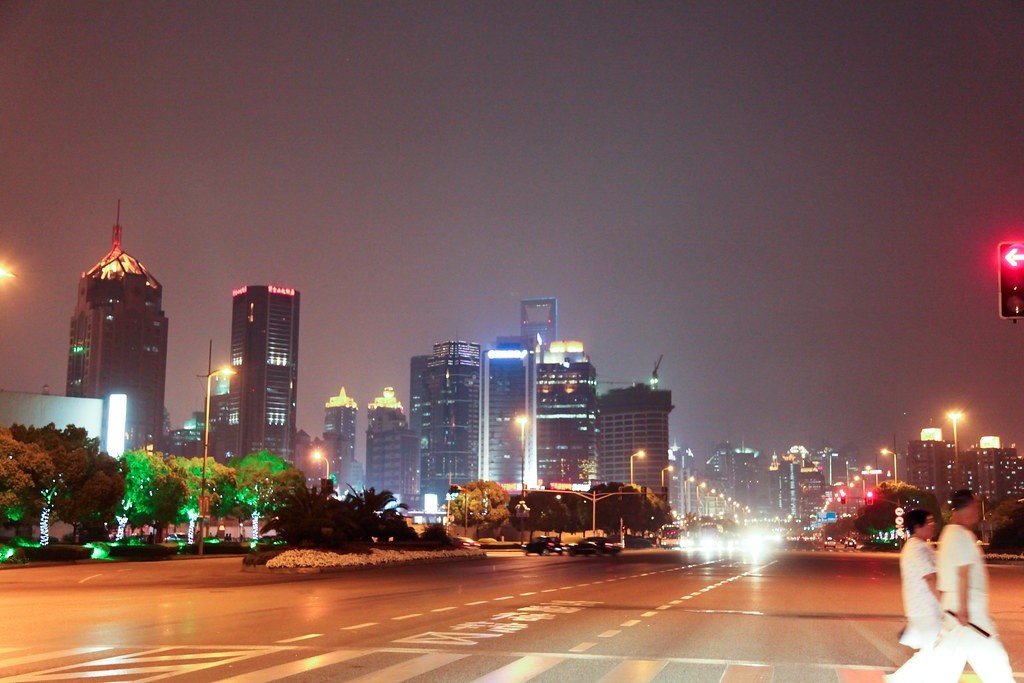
[866,491,873,506]
[841,492,845,504]
[450,484,454,494]
[454,485,458,493]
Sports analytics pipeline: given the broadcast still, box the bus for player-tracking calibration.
[661,525,682,549]
[700,522,723,541]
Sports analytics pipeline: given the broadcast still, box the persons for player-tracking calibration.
[225,534,232,542]
[239,534,243,542]
[147,532,153,544]
[899,489,1017,683]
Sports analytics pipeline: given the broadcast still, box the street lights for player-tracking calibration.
[312,451,329,501]
[517,415,526,546]
[630,451,751,525]
[949,412,961,490]
[880,449,897,484]
[198,368,232,558]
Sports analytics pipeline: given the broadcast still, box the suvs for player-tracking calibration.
[521,536,562,556]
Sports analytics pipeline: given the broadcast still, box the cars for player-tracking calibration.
[840,536,848,544]
[568,537,619,557]
[453,536,481,550]
[824,537,836,549]
[164,533,188,543]
[844,538,857,549]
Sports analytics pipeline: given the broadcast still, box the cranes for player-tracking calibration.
[652,354,664,391]
